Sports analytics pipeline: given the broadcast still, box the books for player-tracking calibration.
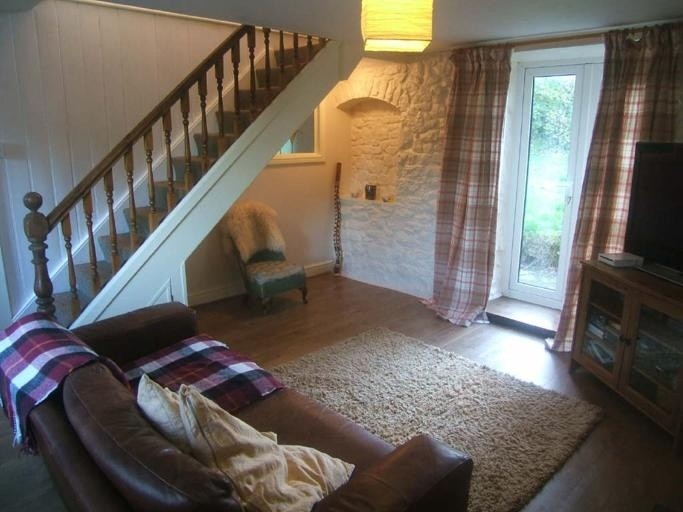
[584,312,680,371]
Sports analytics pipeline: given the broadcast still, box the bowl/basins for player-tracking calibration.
[380,194,394,202]
[351,192,361,198]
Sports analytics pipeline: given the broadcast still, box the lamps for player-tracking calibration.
[359,2,433,54]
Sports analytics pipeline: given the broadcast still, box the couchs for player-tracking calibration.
[5,299,471,512]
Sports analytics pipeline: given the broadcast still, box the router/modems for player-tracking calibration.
[598,252,645,268]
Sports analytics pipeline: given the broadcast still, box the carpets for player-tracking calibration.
[264,325,607,511]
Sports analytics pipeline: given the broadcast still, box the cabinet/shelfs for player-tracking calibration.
[568,252,683,449]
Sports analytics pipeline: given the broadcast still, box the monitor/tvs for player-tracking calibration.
[622,142,683,285]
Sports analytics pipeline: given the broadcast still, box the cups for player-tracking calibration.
[365,184,376,200]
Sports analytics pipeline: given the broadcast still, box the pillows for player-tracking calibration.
[136,373,354,511]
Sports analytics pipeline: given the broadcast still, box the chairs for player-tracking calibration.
[214,202,309,316]
